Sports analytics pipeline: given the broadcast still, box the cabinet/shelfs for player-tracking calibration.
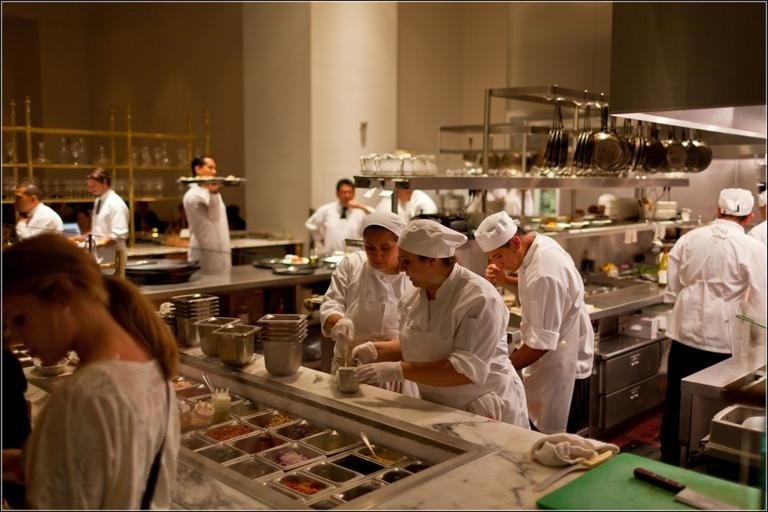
[3,96,211,245]
[352,84,691,318]
[599,342,660,431]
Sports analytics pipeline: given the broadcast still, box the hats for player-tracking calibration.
[719,188,754,217]
[758,190,767,207]
[474,211,517,253]
[397,220,467,258]
[362,211,407,242]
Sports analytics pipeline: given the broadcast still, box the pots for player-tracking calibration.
[624,118,647,172]
[574,102,593,168]
[683,127,698,169]
[543,98,568,167]
[665,126,687,170]
[692,128,712,171]
[645,121,664,174]
[595,108,631,171]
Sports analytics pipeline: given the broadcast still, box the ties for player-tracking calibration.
[97,200,100,214]
[342,208,347,218]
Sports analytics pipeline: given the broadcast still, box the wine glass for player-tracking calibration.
[118,177,164,199]
[39,177,89,199]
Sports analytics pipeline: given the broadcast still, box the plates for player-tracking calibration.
[591,221,611,225]
[31,362,68,375]
[279,257,309,266]
[654,201,677,219]
[570,222,590,228]
[540,221,570,232]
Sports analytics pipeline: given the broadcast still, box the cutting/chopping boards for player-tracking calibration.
[536,453,763,512]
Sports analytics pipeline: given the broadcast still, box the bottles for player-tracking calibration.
[658,247,670,285]
[464,137,476,167]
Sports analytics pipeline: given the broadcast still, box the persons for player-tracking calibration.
[74,166,130,276]
[13,180,64,243]
[3,232,182,510]
[135,155,246,275]
[318,209,595,434]
[747,189,767,246]
[77,209,91,234]
[303,179,437,264]
[2,346,34,509]
[658,187,767,466]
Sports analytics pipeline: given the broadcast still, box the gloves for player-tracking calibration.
[353,361,405,384]
[352,342,378,367]
[331,318,354,344]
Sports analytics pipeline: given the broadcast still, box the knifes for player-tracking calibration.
[533,451,612,493]
[633,466,743,511]
